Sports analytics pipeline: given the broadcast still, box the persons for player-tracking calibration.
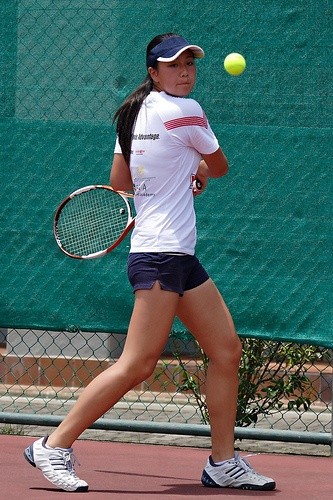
[22,33,276,492]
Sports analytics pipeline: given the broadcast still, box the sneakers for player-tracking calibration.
[23,436,88,492]
[201,455,275,490]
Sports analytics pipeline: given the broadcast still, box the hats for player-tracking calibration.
[146,37,205,66]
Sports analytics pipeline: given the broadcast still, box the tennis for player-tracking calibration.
[224,52,246,76]
